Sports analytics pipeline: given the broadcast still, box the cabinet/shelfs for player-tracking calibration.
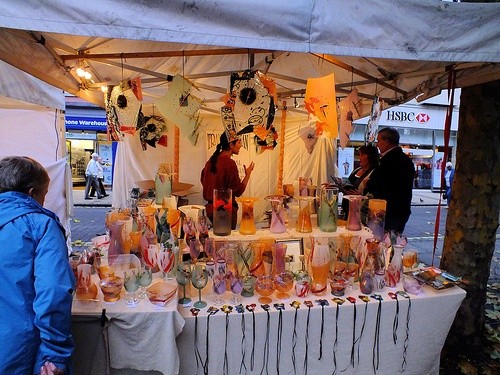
[72,224,466,375]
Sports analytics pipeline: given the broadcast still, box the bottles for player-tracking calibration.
[107,222,125,266]
[143,235,160,273]
[224,233,403,297]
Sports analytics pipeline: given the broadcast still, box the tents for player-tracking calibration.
[0,59,74,257]
[0,0,500,215]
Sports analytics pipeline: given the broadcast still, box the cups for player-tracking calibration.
[69,256,83,281]
[328,275,348,297]
[77,264,91,284]
[213,176,387,243]
[76,281,98,303]
[404,272,426,295]
[98,276,122,302]
[94,257,115,279]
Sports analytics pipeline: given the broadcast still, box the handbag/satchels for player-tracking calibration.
[443,192,447,199]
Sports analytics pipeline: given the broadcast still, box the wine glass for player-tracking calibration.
[403,250,419,271]
[123,217,293,308]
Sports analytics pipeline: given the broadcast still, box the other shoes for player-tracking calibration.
[85,197,93,200]
[90,195,96,197]
[98,195,104,199]
[104,193,110,196]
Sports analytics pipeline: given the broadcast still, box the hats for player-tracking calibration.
[91,153,98,158]
[446,162,452,167]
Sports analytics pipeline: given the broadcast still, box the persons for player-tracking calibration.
[0,155,75,375]
[340,128,455,239]
[84,153,110,200]
[200,130,255,230]
